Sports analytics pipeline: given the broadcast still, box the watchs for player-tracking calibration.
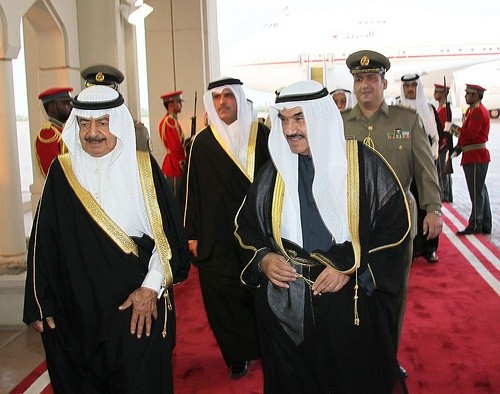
[433,210,442,217]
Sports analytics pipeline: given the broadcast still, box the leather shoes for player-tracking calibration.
[424,250,439,262]
[230,361,248,378]
[442,198,454,203]
[396,365,408,379]
[457,227,481,235]
[480,228,492,235]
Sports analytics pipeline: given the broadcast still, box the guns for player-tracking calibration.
[189,90,196,137]
[443,76,455,175]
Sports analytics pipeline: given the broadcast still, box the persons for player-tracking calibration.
[181,76,271,378]
[228,83,412,394]
[339,50,443,378]
[159,90,186,219]
[35,88,74,178]
[330,89,353,113]
[390,74,450,263]
[444,84,491,235]
[433,83,454,204]
[22,86,191,394]
[80,64,153,153]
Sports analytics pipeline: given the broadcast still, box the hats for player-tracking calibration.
[38,87,75,104]
[345,50,391,75]
[80,65,124,92]
[160,90,186,104]
[465,84,486,96]
[433,84,450,93]
